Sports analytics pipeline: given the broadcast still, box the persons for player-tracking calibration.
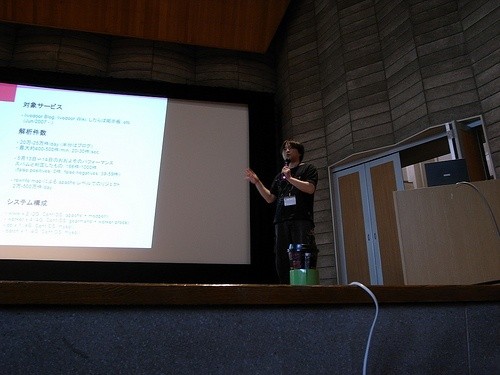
[245,140,317,285]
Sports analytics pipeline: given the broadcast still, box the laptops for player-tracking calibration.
[425,159,470,187]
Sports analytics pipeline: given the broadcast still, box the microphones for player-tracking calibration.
[283,158,291,179]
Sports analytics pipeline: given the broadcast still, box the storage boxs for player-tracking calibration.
[289,268,320,285]
